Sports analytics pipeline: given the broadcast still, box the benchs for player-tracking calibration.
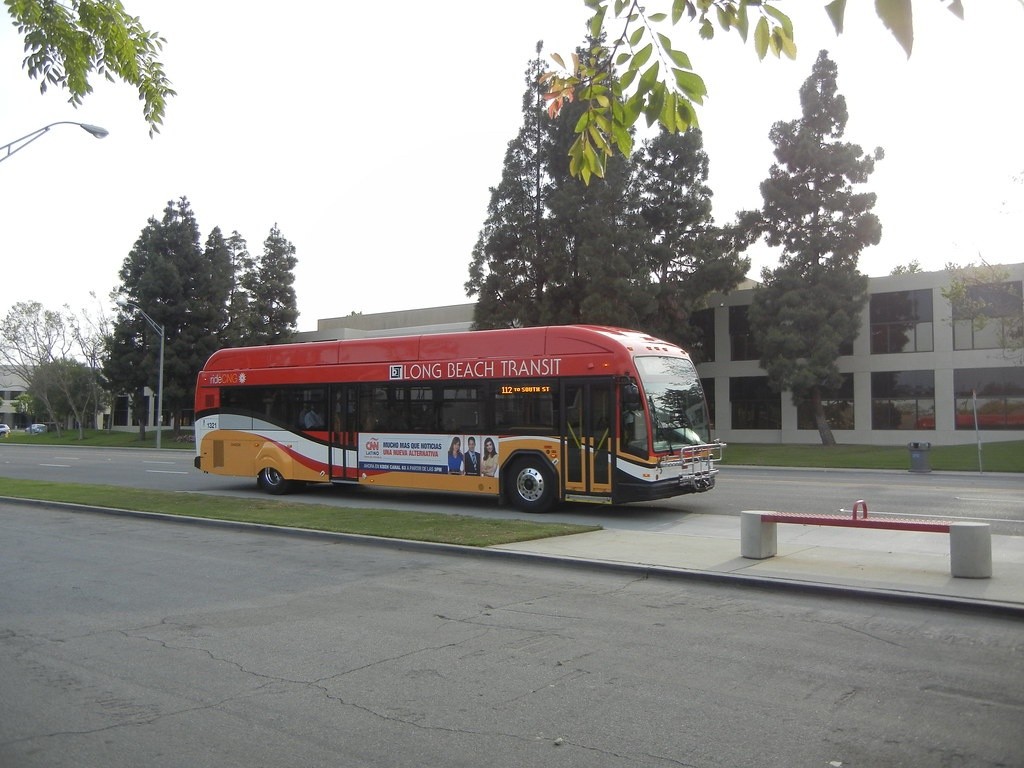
[740,501,992,579]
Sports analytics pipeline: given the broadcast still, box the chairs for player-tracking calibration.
[299,408,310,427]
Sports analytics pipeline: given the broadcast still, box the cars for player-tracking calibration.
[24,423,47,434]
[0,423,11,435]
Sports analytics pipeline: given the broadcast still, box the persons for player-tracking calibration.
[299,385,348,430]
[622,410,634,449]
[448,437,464,475]
[464,436,481,476]
[481,437,498,477]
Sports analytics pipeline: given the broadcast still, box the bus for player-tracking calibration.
[192,323,726,513]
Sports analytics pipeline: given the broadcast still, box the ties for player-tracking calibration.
[472,455,477,471]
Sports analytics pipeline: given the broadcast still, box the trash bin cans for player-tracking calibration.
[908,441,932,472]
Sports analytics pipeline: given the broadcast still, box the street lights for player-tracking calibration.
[116,299,164,448]
